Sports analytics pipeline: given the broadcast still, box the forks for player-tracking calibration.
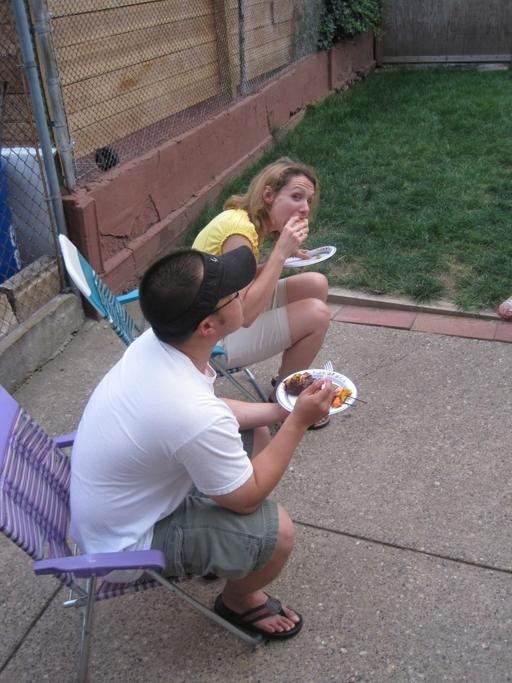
[324,360,333,388]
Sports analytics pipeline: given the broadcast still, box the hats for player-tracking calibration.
[149,244,259,336]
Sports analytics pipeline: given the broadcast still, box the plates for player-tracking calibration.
[276,367,358,417]
[281,244,337,269]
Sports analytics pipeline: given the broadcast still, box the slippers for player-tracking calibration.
[214,586,304,641]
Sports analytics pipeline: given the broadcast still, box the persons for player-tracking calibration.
[189,157,330,430]
[60,245,334,641]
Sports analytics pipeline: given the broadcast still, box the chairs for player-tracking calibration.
[57,229,277,409]
[0,382,267,682]
